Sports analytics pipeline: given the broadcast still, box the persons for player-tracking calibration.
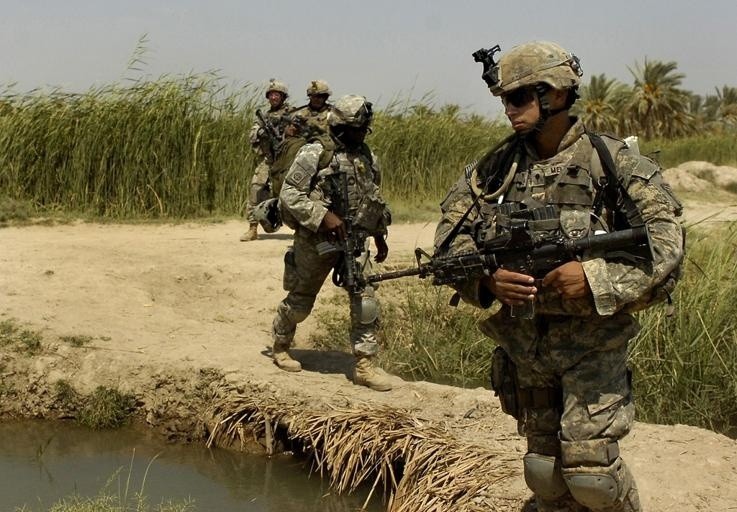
[284,80,332,144]
[271,94,393,391]
[433,41,687,512]
[240,82,296,241]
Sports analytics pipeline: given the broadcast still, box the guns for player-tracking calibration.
[281,113,324,139]
[312,172,363,313]
[366,221,655,319]
[256,107,285,144]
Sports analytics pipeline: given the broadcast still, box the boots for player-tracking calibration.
[345,354,393,392]
[271,333,302,373]
[240,222,258,241]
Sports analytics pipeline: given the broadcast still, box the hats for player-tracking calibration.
[324,93,374,128]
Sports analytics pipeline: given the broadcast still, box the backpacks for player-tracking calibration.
[271,135,308,230]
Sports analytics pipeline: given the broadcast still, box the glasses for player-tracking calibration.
[494,83,561,110]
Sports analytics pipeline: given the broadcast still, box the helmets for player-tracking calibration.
[489,40,583,98]
[265,78,289,99]
[306,79,333,96]
